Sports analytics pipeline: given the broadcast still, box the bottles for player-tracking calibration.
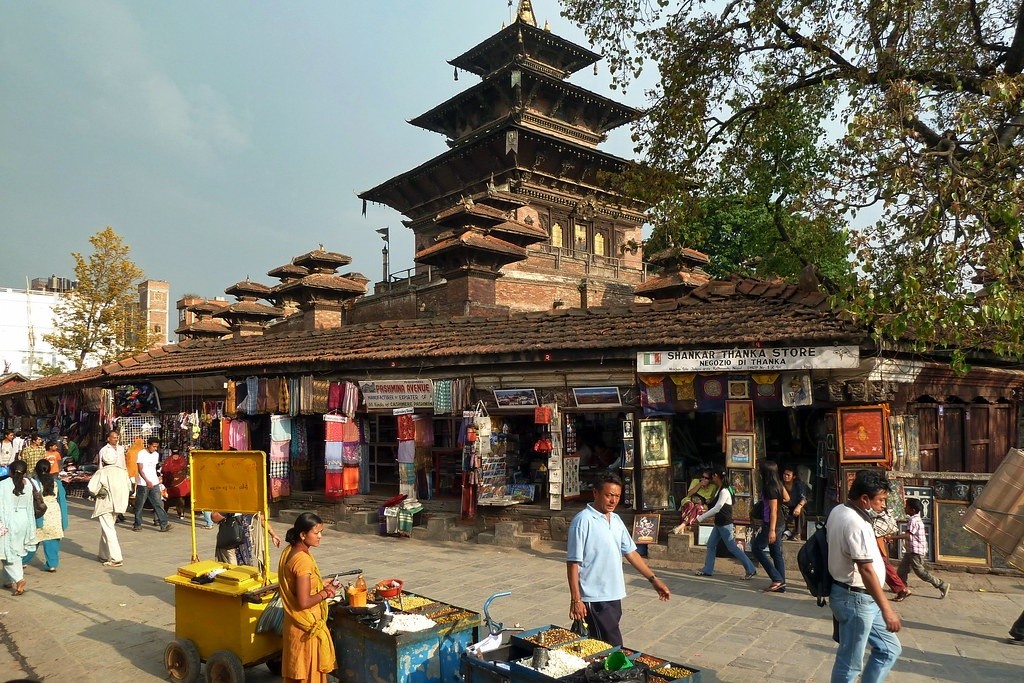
[356,575,366,588]
[346,583,354,593]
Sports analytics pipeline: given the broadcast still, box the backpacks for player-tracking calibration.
[797,503,841,608]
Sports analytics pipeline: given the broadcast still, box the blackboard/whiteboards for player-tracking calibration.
[932,498,993,566]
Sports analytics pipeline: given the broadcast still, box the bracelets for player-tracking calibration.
[571,598,582,603]
[648,575,657,583]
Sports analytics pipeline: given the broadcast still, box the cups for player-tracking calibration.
[604,651,634,672]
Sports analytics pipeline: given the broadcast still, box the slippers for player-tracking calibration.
[1006,636,1024,646]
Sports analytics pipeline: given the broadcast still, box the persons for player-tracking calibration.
[566,468,670,647]
[672,460,811,594]
[1008,611,1024,646]
[211,512,282,568]
[0,430,215,597]
[886,498,951,599]
[278,513,341,683]
[585,444,622,475]
[873,511,912,602]
[825,472,902,683]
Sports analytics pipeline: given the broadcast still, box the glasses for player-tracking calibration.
[700,475,710,480]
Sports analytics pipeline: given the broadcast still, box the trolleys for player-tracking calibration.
[163,561,285,683]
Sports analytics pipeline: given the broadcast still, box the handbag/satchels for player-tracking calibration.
[870,508,899,538]
[691,492,708,506]
[256,586,283,636]
[750,493,764,519]
[216,512,246,549]
[27,477,47,519]
[466,399,554,457]
[158,399,225,457]
[570,619,588,636]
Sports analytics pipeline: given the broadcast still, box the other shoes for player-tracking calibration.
[792,534,800,541]
[694,565,950,601]
[679,527,684,534]
[98,554,108,563]
[673,526,680,535]
[780,534,792,541]
[111,508,215,533]
[102,560,123,567]
[22,564,27,568]
[46,567,56,573]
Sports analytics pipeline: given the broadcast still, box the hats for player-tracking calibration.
[102,448,118,465]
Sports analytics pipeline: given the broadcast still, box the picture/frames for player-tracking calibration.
[841,465,935,562]
[632,514,660,544]
[573,388,623,408]
[725,380,756,497]
[836,403,889,463]
[638,418,674,509]
[492,389,541,409]
[932,499,992,568]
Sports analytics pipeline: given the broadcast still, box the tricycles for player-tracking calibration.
[457,590,722,683]
[322,569,483,682]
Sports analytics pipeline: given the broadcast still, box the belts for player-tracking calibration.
[832,579,870,595]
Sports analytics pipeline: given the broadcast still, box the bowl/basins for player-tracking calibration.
[375,579,403,598]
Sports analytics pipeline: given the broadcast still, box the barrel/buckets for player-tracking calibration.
[347,588,368,606]
